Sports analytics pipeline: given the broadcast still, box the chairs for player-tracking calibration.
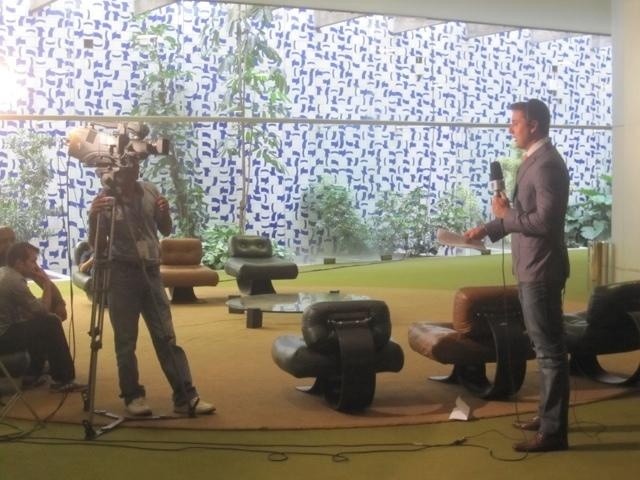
[159,237,220,304]
[407,285,536,401]
[224,235,298,295]
[561,279,639,387]
[71,240,107,304]
[270,300,404,416]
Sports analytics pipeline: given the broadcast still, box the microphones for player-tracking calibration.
[489,161,509,198]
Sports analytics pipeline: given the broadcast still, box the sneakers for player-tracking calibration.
[173,397,216,414]
[21,374,51,390]
[49,379,88,393]
[125,397,152,415]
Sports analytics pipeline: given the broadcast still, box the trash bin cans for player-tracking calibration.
[588,241,616,293]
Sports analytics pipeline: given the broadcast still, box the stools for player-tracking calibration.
[1,348,43,426]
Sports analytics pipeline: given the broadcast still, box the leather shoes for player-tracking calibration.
[512,415,541,430]
[513,432,568,451]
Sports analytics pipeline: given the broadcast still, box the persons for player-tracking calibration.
[87,162,214,414]
[464,98,570,450]
[79,250,95,272]
[0,242,76,389]
